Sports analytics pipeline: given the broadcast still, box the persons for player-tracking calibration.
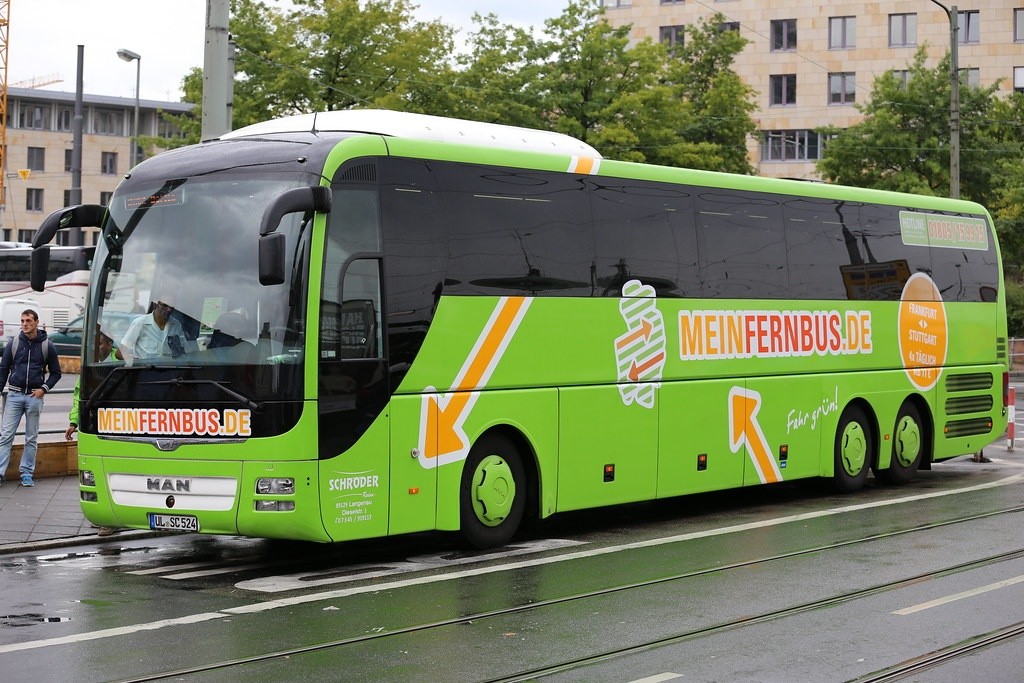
[0,309,61,487]
[66,331,119,440]
[116,290,187,364]
[207,296,253,349]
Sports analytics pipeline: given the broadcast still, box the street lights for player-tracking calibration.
[116,47,142,166]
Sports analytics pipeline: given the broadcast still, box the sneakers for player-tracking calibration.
[20,476,34,486]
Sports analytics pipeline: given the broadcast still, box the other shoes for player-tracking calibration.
[98,526,116,535]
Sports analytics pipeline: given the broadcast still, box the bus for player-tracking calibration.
[1,238,96,368]
[27,107,1012,553]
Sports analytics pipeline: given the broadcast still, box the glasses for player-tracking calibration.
[158,300,175,309]
